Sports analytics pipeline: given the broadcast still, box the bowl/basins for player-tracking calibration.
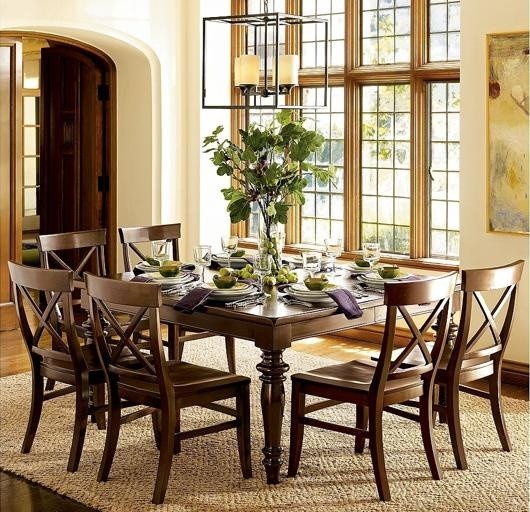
[147,256,160,266]
[304,278,327,291]
[214,275,237,288]
[231,250,245,257]
[378,267,399,278]
[159,267,179,277]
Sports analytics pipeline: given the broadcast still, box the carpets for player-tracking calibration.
[0,329,529,512]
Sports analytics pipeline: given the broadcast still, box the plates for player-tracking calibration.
[136,261,160,272]
[358,272,414,283]
[201,283,258,300]
[345,263,395,272]
[213,252,229,263]
[287,255,304,268]
[142,272,194,289]
[283,283,340,303]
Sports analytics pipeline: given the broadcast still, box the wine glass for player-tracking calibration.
[254,254,271,293]
[272,232,285,264]
[221,236,238,267]
[363,243,380,272]
[324,238,341,276]
[152,241,170,266]
[194,245,211,282]
[303,252,321,277]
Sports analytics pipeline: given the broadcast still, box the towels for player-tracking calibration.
[327,288,364,319]
[181,263,195,271]
[175,287,216,314]
[398,274,421,283]
[242,257,254,264]
[129,275,153,284]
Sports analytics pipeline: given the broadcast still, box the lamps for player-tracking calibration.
[201,0,327,107]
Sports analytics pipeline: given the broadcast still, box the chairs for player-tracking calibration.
[371,260,525,469]
[4,260,154,472]
[36,229,153,388]
[118,223,237,375]
[290,272,457,501]
[84,273,254,502]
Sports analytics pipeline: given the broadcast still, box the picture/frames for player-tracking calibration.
[487,32,530,236]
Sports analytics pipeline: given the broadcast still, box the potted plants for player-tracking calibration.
[201,111,342,269]
